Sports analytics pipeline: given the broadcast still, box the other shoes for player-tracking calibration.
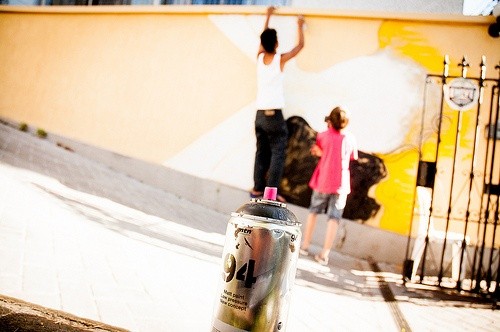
[314,253,328,265]
[299,248,309,256]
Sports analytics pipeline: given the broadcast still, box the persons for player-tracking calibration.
[249,3,307,205]
[301,106,358,266]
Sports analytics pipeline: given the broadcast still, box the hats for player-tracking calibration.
[330,107,348,130]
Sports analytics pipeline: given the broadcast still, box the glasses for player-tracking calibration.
[325,116,331,122]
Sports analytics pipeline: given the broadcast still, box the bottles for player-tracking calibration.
[208,184,302,332]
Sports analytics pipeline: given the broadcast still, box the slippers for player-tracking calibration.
[251,192,263,198]
[276,194,286,203]
